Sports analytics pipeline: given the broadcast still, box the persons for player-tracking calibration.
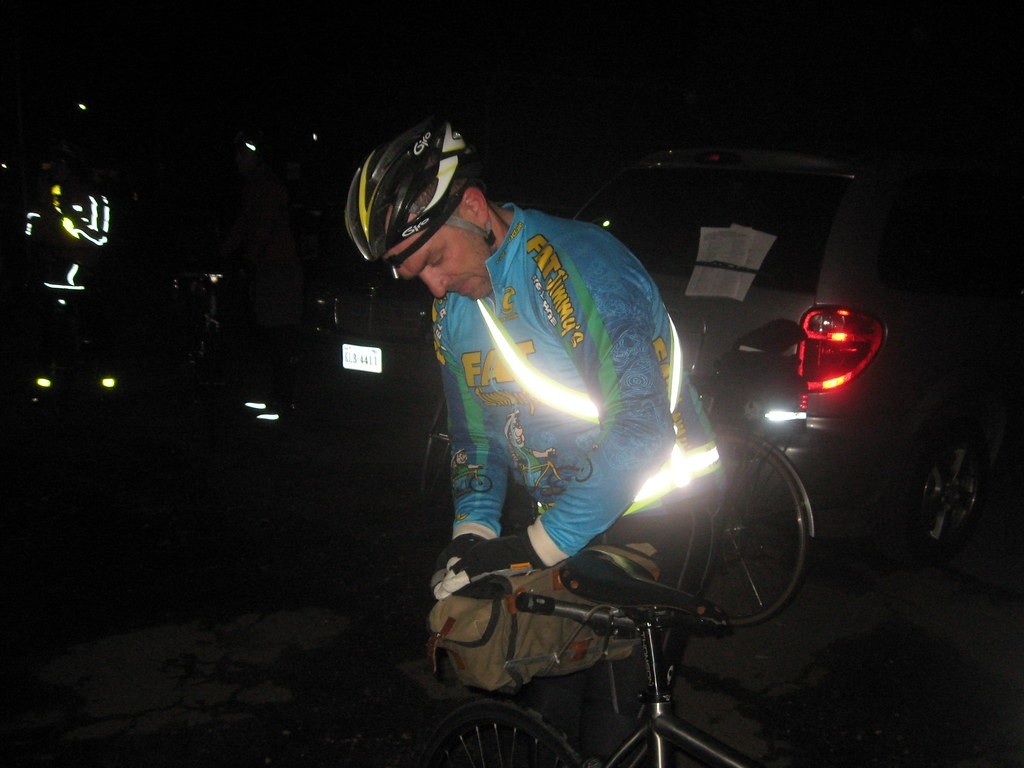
[210,129,306,409]
[346,117,727,768]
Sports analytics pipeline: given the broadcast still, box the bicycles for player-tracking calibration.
[412,550,769,768]
[654,424,816,627]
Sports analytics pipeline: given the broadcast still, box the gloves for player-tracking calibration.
[432,527,548,601]
[429,533,488,587]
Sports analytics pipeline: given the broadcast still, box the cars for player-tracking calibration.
[570,146,1024,570]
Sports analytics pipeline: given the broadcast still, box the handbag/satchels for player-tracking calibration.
[425,543,673,690]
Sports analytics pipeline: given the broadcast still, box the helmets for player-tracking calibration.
[343,115,487,268]
